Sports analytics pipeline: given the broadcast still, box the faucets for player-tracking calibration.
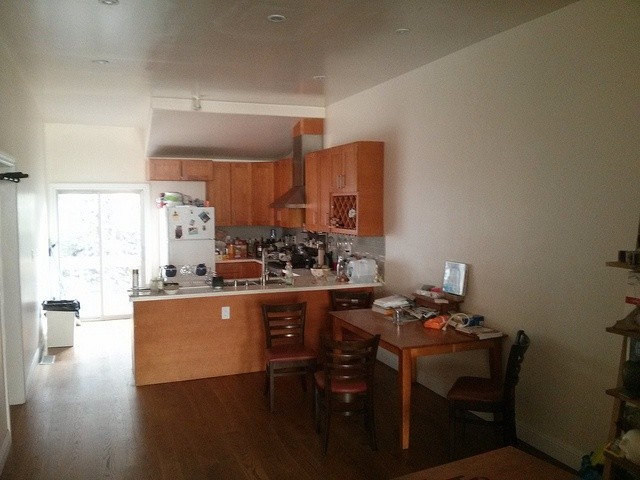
[262,248,267,286]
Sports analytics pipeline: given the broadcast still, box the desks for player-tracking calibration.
[327,308,508,449]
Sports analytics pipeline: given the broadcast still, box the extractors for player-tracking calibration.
[269,186,307,209]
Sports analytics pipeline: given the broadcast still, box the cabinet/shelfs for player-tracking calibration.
[602,222,640,480]
[206,161,252,227]
[275,160,303,228]
[146,159,213,181]
[303,142,383,237]
[253,162,276,226]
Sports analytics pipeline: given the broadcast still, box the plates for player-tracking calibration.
[163,290,179,294]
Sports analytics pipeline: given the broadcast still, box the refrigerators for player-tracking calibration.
[159,205,216,280]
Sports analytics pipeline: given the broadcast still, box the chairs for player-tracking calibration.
[313,327,381,454]
[447,330,531,462]
[260,301,318,415]
[326,290,375,311]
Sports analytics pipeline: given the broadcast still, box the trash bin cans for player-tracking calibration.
[42,300,78,348]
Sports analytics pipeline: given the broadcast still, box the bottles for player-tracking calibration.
[318,246,325,266]
[261,237,264,247]
[336,257,344,277]
[284,262,294,286]
[248,239,253,256]
[257,242,262,257]
[132,269,139,291]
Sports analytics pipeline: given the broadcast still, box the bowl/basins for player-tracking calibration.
[310,268,331,279]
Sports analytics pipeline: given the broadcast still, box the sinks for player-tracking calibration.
[223,278,285,286]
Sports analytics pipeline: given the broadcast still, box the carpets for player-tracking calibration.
[396,445,578,480]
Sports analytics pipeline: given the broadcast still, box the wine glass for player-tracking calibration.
[342,234,349,248]
[349,234,355,247]
[337,234,343,248]
